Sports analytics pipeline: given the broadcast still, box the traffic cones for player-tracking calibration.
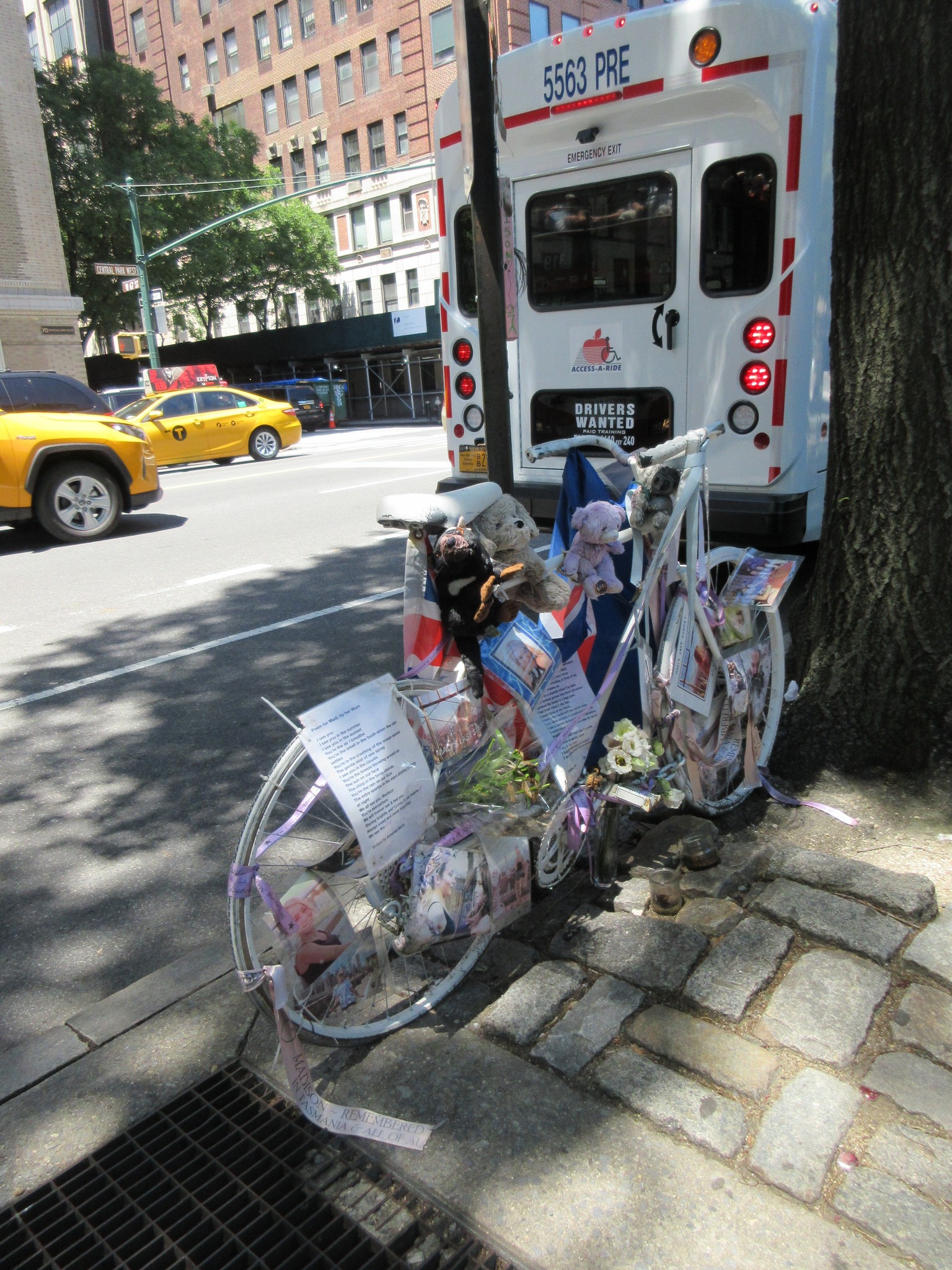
[328,410,335,429]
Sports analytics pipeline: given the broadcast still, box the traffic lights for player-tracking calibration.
[113,335,141,356]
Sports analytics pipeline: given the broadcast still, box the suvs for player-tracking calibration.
[248,384,326,433]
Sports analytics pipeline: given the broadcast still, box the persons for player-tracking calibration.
[746,648,765,698]
[427,860,457,939]
[454,860,491,939]
[453,701,482,753]
[512,845,525,905]
[283,897,355,983]
[737,563,782,604]
[505,638,553,689]
[726,661,744,694]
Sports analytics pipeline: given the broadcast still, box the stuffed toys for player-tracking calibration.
[471,493,571,614]
[431,527,520,699]
[562,501,627,599]
[628,463,681,565]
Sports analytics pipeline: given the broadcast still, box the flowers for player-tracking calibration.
[432,728,550,818]
[598,718,685,882]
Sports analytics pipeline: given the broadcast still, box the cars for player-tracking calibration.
[112,364,302,467]
[0,369,163,545]
[97,387,145,413]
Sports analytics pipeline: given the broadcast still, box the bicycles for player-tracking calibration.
[219,426,786,1079]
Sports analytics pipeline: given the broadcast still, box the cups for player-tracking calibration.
[680,829,719,867]
[648,868,682,915]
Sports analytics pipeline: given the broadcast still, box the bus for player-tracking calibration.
[431,0,829,556]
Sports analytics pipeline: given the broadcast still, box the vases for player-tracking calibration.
[594,806,617,890]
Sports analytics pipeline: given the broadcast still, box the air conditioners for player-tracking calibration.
[347,179,362,194]
[201,84,215,98]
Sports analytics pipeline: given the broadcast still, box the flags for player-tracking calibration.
[404,449,649,770]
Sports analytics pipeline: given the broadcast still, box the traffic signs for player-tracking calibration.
[138,288,163,307]
